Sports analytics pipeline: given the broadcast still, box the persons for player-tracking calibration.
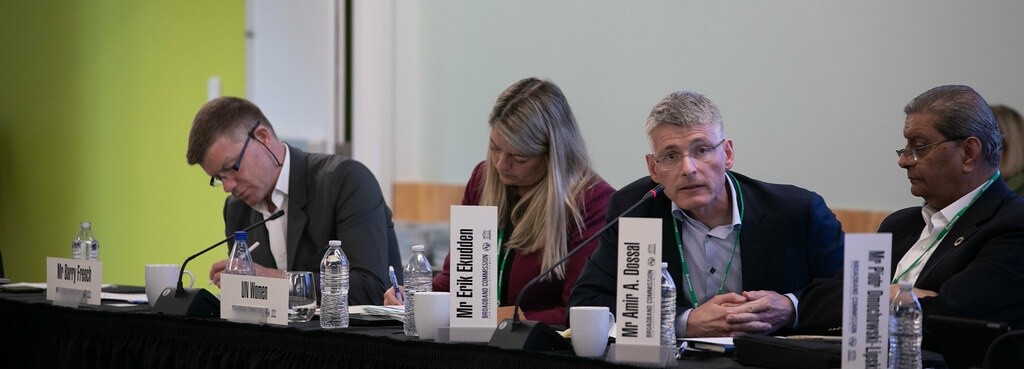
[384,77,618,326]
[988,105,1024,199]
[186,96,404,306]
[565,93,845,338]
[877,85,1024,318]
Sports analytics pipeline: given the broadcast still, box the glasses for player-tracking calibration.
[210,120,260,187]
[896,138,954,164]
[651,139,726,170]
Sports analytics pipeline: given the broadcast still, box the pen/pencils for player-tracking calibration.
[388,266,404,305]
[208,241,261,285]
[676,341,688,360]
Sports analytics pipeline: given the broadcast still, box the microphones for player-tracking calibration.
[154,209,285,318]
[487,184,667,352]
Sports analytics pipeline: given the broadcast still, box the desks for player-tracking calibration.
[0,282,756,369]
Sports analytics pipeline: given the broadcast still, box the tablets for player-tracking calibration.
[925,315,1011,369]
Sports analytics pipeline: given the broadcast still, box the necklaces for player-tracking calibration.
[891,168,1000,283]
[498,224,512,306]
[672,170,744,308]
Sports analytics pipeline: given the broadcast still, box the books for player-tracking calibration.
[695,342,735,353]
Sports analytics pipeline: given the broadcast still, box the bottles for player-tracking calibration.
[72,222,99,261]
[225,231,256,277]
[889,281,922,369]
[660,262,676,348]
[320,240,350,329]
[404,245,433,336]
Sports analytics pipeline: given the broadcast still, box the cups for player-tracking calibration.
[414,292,451,339]
[283,273,317,322]
[145,264,194,308]
[570,306,614,357]
